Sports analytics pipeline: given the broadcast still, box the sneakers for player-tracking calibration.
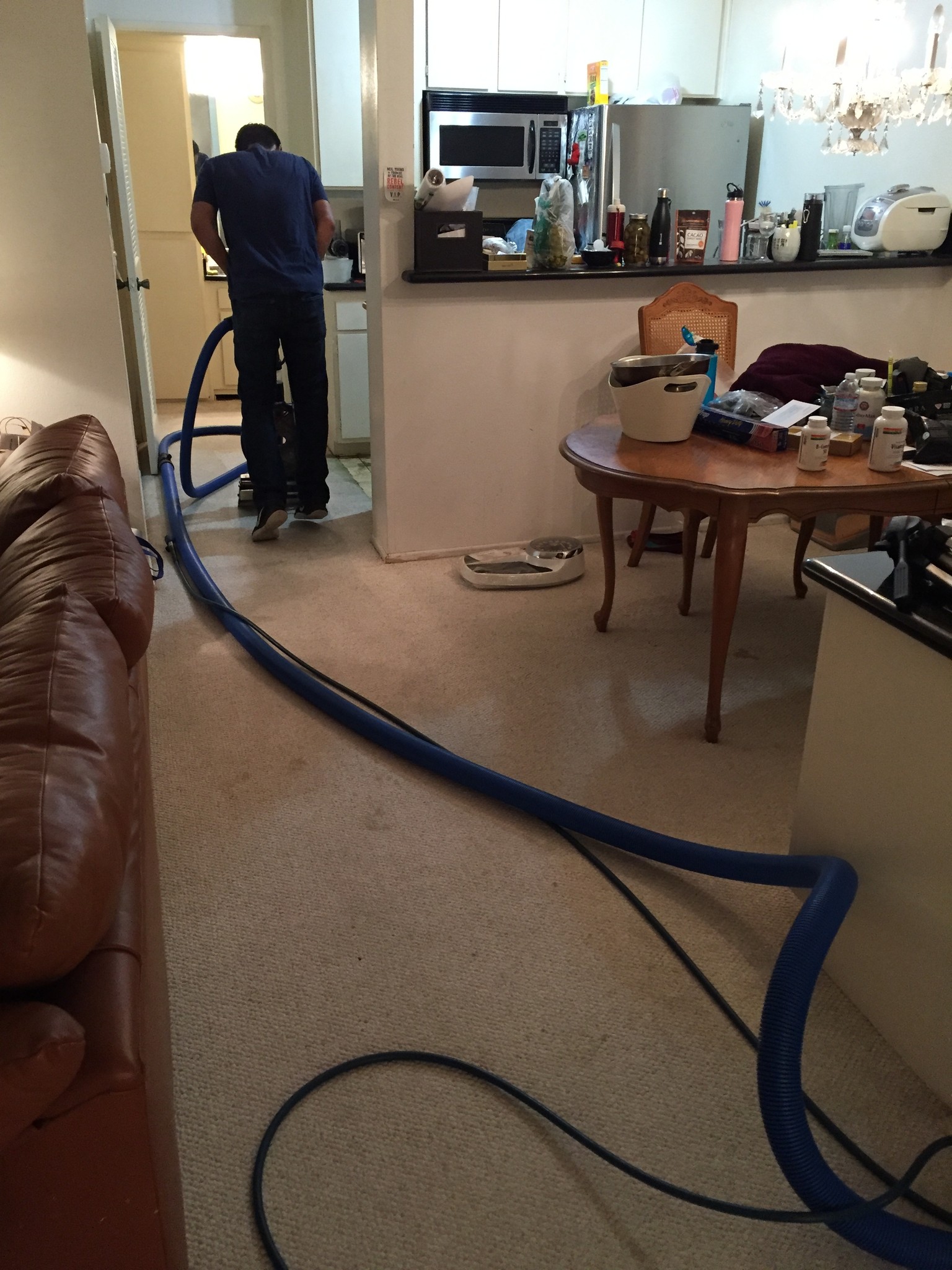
[294,505,327,519]
[252,503,288,541]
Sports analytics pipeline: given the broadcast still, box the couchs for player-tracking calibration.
[1,413,188,1270]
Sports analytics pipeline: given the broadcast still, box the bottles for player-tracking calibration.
[828,226,852,251]
[830,372,860,434]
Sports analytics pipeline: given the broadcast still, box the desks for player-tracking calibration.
[557,411,951,746]
[786,538,952,1116]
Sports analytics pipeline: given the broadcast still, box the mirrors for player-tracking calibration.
[182,35,267,267]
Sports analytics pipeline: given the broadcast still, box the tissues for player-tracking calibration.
[580,238,613,270]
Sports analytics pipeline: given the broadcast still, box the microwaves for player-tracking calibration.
[422,91,568,185]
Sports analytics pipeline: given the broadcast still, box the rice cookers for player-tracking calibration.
[852,184,952,258]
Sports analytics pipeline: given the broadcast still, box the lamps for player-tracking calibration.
[754,0,952,160]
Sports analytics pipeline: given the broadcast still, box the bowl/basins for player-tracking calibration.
[610,353,712,391]
[607,354,711,443]
[579,250,615,268]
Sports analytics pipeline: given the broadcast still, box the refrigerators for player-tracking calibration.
[572,100,752,258]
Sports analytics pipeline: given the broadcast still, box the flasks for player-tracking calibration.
[605,197,627,266]
[798,191,823,262]
[649,186,672,265]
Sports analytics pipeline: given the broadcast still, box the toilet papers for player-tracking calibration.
[415,168,446,208]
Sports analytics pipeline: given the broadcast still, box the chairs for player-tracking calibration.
[628,281,817,616]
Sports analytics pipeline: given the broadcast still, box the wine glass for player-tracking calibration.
[755,210,777,263]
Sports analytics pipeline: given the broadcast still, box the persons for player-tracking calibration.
[191,122,339,541]
[192,140,210,184]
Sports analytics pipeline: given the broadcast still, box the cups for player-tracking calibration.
[720,183,744,262]
[770,226,800,262]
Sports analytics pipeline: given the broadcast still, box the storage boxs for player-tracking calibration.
[413,210,485,274]
